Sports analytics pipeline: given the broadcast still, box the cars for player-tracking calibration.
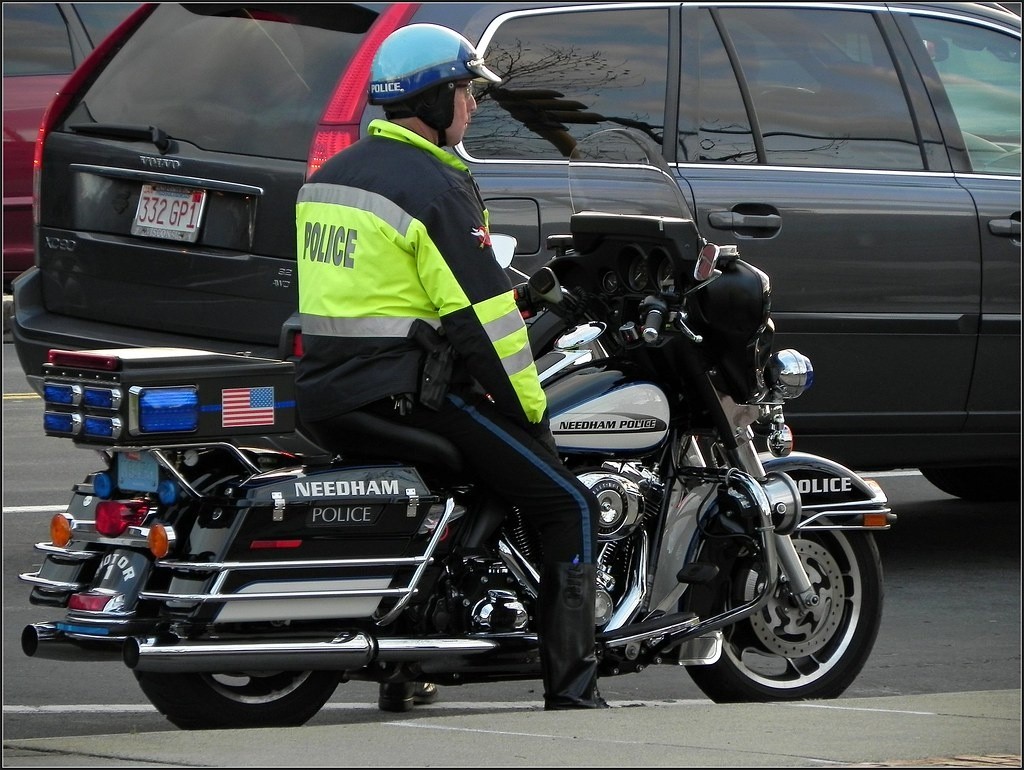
[1,2,144,344]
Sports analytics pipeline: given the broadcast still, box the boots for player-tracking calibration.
[377,676,440,713]
[538,561,616,710]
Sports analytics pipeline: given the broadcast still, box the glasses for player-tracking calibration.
[456,80,473,100]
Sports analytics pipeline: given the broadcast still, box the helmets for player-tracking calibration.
[366,22,501,105]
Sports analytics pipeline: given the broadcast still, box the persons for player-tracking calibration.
[288,22,612,711]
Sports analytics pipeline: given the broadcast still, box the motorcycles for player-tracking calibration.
[23,133,894,732]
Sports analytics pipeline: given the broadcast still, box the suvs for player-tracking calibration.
[12,2,1024,501]
